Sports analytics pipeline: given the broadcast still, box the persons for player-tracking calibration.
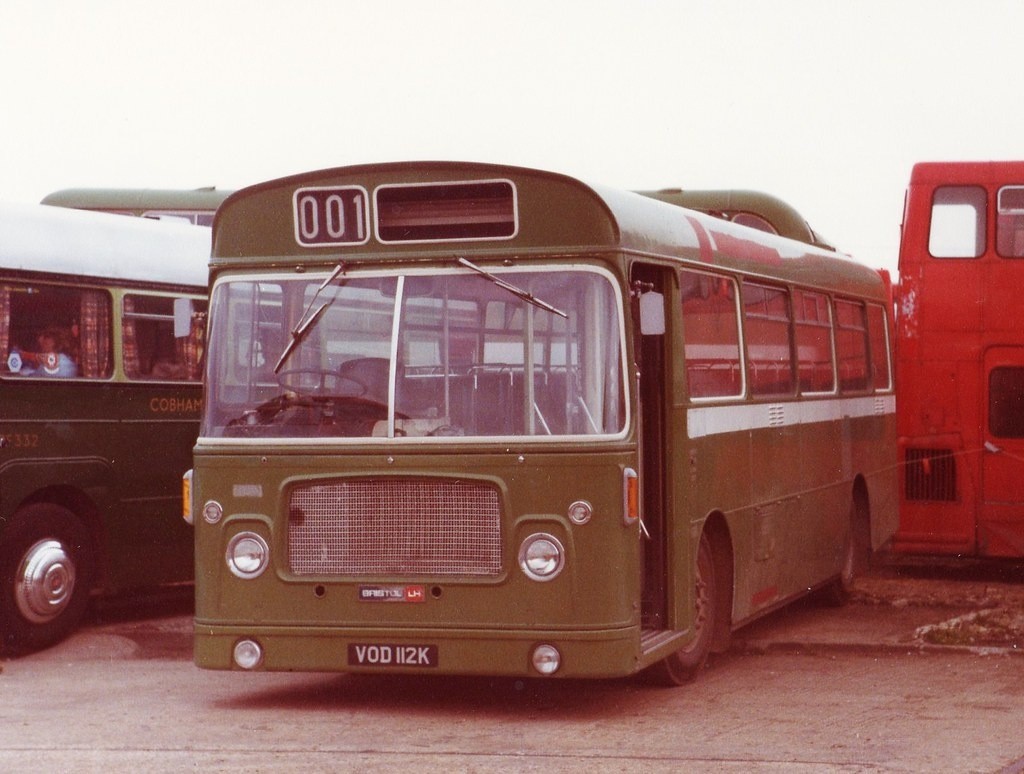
[11,323,76,377]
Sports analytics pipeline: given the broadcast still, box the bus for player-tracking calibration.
[182,160,899,688]
[0,198,620,659]
[880,162,1024,571]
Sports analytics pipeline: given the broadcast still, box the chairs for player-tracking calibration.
[336,355,406,409]
[431,361,824,436]
